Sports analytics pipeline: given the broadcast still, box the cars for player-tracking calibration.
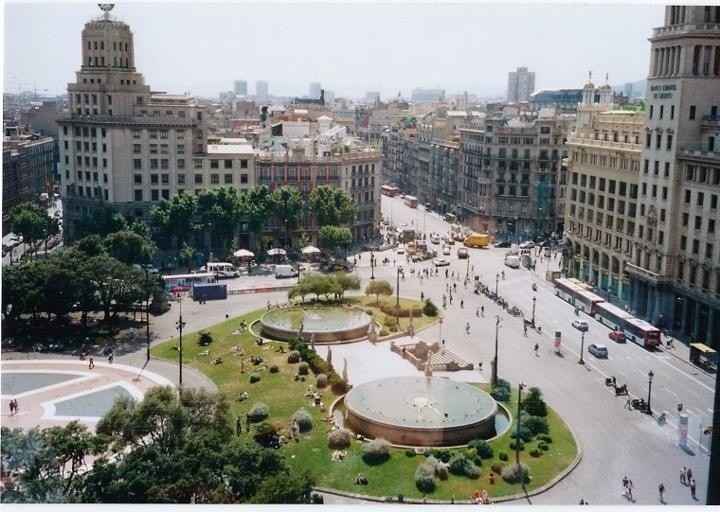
[572,320,588,331]
[397,245,404,253]
[435,259,450,266]
[458,248,468,258]
[589,344,607,359]
[494,241,511,248]
[430,235,455,255]
[608,331,627,343]
[518,241,536,249]
[142,263,159,274]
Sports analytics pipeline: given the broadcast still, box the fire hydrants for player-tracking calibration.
[489,473,495,484]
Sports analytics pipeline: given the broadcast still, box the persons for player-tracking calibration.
[521,243,561,268]
[575,305,579,315]
[357,472,364,485]
[580,499,588,505]
[522,325,528,336]
[664,328,672,346]
[422,495,427,504]
[612,376,645,413]
[9,399,18,416]
[622,476,633,499]
[199,294,207,304]
[533,283,536,291]
[13,315,134,369]
[402,267,523,334]
[625,304,631,314]
[161,251,209,274]
[215,349,263,372]
[236,413,299,449]
[533,344,538,355]
[658,483,665,503]
[354,220,513,267]
[679,466,696,498]
[451,498,455,503]
[474,489,490,504]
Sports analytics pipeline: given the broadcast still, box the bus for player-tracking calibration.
[404,194,419,209]
[593,301,660,349]
[552,278,606,316]
[381,185,398,197]
[161,273,219,289]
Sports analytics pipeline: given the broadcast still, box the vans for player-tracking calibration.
[207,262,240,278]
[275,265,299,278]
[505,256,520,268]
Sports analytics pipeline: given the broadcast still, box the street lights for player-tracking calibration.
[581,326,585,361]
[141,264,150,361]
[176,292,182,383]
[396,267,405,324]
[646,370,654,411]
[496,273,499,298]
[517,383,526,462]
[467,253,470,279]
[533,297,536,326]
[371,251,375,279]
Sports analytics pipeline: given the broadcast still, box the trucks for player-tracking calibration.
[463,235,489,248]
